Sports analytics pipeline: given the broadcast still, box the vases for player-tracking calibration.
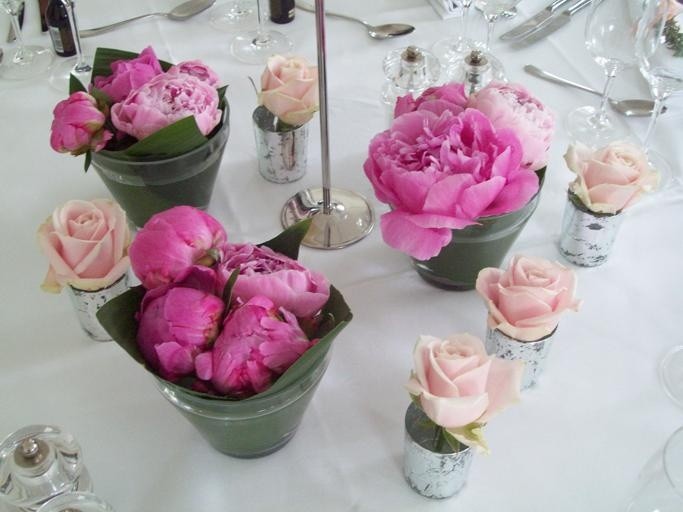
[146,334,333,460]
[248,105,309,182]
[409,186,548,292]
[397,400,472,500]
[63,274,130,346]
[553,191,625,268]
[480,303,553,394]
[79,112,238,220]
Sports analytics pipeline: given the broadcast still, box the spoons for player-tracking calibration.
[297,1,416,41]
[75,0,215,37]
[520,62,668,119]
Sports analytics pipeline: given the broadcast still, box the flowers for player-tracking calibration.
[250,52,321,127]
[475,253,590,342]
[365,80,553,260]
[43,38,221,160]
[406,327,517,452]
[118,213,337,390]
[30,184,137,294]
[564,137,660,214]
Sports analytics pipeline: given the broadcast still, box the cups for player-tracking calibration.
[250,106,309,184]
[147,339,334,466]
[396,168,545,291]
[401,400,475,503]
[556,196,625,268]
[623,426,683,511]
[484,320,559,390]
[70,94,231,237]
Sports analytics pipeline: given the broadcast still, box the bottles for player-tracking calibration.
[0,425,112,512]
[45,1,80,58]
[381,46,506,108]
[267,0,295,25]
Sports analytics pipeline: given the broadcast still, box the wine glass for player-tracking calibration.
[428,0,520,68]
[563,0,682,186]
[0,0,96,96]
[208,0,297,67]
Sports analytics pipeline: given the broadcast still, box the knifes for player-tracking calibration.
[501,0,592,53]
